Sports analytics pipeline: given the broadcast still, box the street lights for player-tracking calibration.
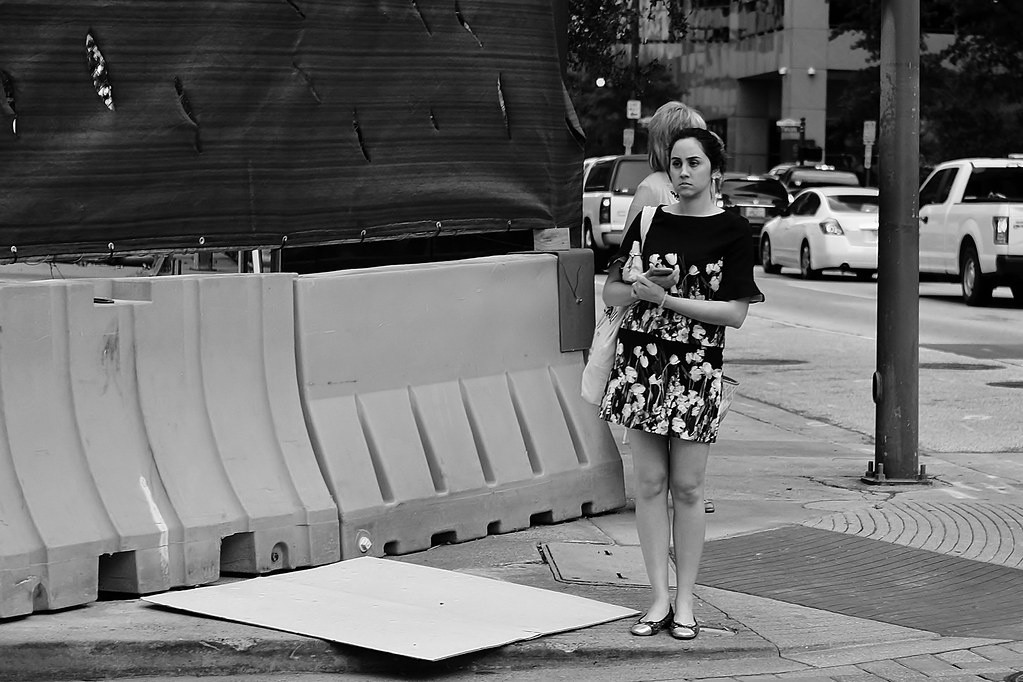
[778,119,806,166]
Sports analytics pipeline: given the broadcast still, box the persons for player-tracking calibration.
[598,127,765,640]
[620,101,714,514]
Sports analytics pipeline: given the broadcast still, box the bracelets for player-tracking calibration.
[658,292,668,308]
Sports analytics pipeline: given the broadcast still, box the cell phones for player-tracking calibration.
[652,268,673,276]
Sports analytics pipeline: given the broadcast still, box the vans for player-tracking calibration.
[770,166,858,195]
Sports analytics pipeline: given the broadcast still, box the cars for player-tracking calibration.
[759,185,880,279]
[716,173,795,233]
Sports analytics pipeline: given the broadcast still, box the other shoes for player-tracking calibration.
[669,613,700,640]
[630,603,675,636]
[704,499,715,512]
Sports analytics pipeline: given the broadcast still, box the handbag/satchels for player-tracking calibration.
[580,205,658,407]
[719,375,740,424]
[622,241,643,284]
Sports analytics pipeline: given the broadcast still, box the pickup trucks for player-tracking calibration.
[918,155,1023,305]
[583,154,666,256]
